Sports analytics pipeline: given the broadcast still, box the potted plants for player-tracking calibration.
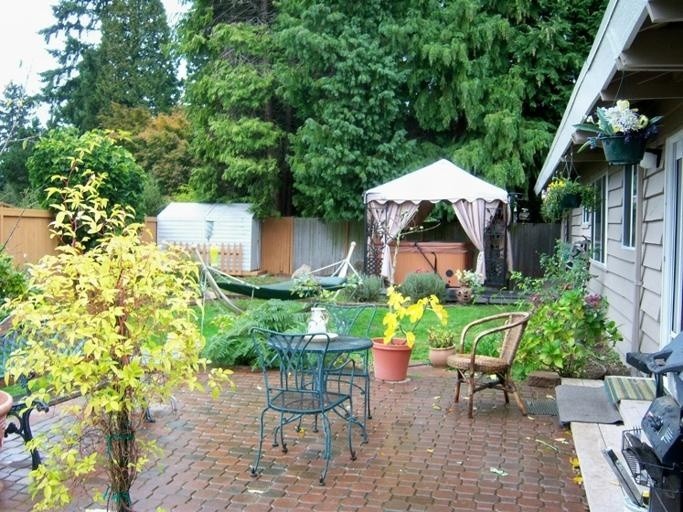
[427,324,455,369]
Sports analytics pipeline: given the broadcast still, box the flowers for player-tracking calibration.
[454,269,484,285]
[383,286,457,350]
[540,97,663,227]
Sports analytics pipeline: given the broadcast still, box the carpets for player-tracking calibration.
[526,376,658,424]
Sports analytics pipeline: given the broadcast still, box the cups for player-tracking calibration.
[306,308,326,336]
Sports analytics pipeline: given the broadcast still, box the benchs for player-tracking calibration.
[1,311,157,469]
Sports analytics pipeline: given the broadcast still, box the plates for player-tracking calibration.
[304,333,337,343]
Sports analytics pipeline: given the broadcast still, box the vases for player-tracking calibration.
[456,284,475,306]
[371,338,411,381]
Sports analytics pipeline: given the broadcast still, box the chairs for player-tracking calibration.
[446,311,530,419]
[247,305,377,486]
